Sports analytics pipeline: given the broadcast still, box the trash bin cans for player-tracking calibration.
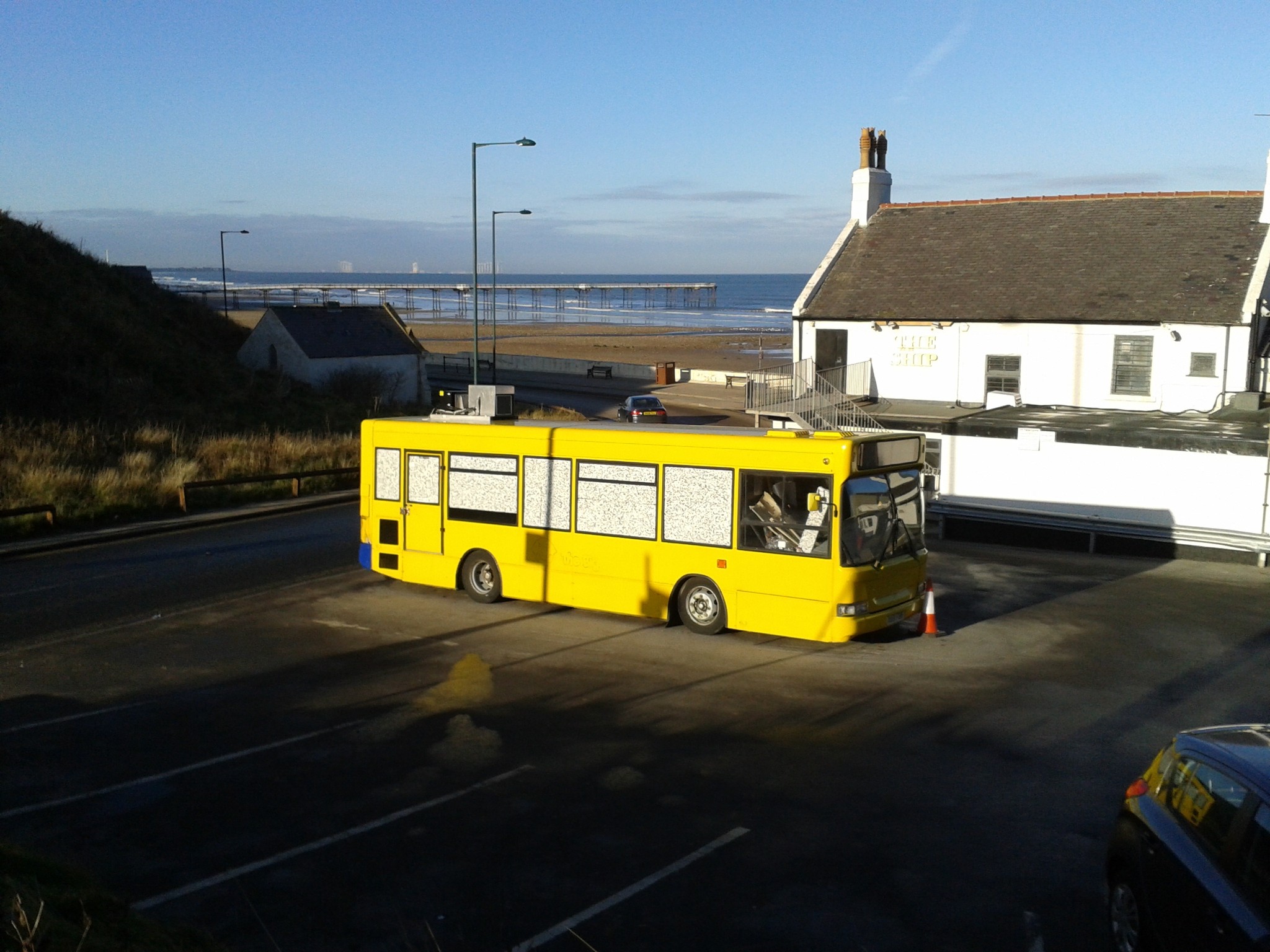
[656,361,675,384]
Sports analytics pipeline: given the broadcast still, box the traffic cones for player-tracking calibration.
[910,584,947,638]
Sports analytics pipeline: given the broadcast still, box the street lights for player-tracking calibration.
[470,138,537,385]
[219,229,251,319]
[490,208,534,384]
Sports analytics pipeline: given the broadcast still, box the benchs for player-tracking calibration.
[726,375,750,388]
[472,359,493,371]
[586,365,613,379]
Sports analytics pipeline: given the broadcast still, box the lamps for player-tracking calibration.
[1171,330,1182,342]
[871,321,876,330]
[931,321,942,329]
[888,322,898,328]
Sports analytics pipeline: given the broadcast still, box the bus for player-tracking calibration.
[357,383,945,644]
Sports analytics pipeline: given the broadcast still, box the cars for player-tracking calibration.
[1098,721,1270,952]
[617,395,668,425]
